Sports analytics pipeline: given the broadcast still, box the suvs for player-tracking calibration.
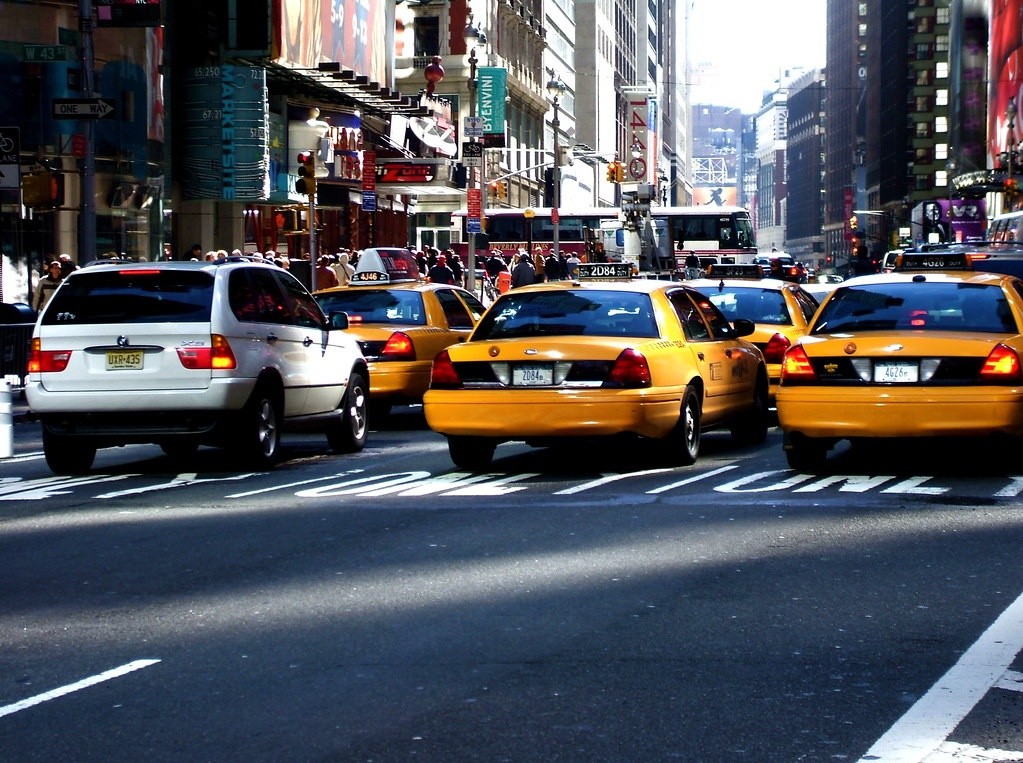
[877,241,1023,278]
[24,256,371,474]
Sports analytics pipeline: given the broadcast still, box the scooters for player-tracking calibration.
[483,274,497,301]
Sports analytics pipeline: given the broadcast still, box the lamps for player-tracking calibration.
[475,22,487,47]
[286,62,434,117]
[465,13,479,38]
[306,108,329,133]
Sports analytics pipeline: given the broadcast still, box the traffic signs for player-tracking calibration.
[51,97,116,119]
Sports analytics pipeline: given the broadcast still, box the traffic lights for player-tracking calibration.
[22,170,65,208]
[607,162,617,183]
[273,208,297,232]
[498,182,508,198]
[295,150,316,194]
[827,256,831,264]
[615,161,627,181]
[489,181,498,197]
[307,207,323,231]
[871,258,876,266]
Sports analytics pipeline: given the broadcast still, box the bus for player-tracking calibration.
[450,204,758,281]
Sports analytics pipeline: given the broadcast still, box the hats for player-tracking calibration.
[439,255,446,262]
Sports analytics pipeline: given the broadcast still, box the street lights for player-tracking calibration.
[630,141,643,181]
[1004,96,1017,179]
[852,206,935,233]
[462,15,487,302]
[945,152,956,242]
[850,214,858,255]
[662,171,669,207]
[546,74,567,261]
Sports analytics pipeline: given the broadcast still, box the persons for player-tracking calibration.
[854,246,877,277]
[407,241,606,295]
[33,245,363,312]
[770,247,809,284]
[685,250,700,279]
[726,232,732,246]
[924,203,955,243]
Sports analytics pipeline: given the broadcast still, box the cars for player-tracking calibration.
[776,252,1023,469]
[423,262,770,468]
[310,248,487,431]
[676,253,844,409]
